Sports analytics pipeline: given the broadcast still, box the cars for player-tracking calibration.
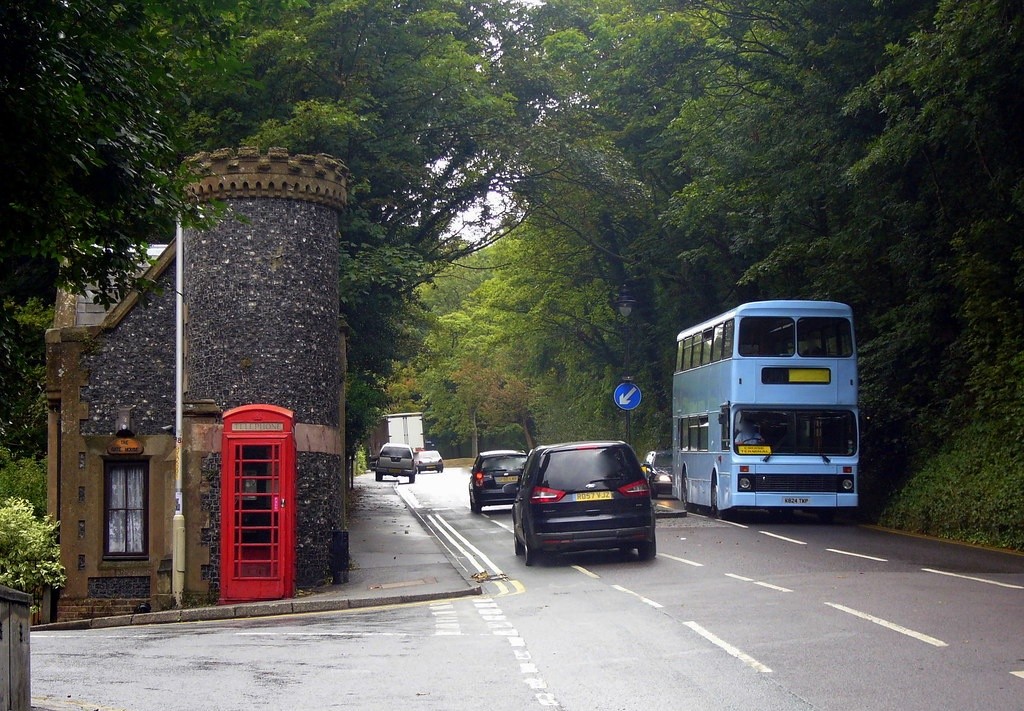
[415,450,444,474]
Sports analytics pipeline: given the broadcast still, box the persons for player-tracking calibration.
[735,419,765,445]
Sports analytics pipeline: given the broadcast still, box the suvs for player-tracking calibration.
[502,439,657,567]
[642,449,673,500]
[373,442,419,484]
[468,448,529,514]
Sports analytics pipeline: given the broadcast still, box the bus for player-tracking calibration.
[672,299,860,524]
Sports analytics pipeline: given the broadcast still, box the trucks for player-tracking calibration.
[369,412,425,471]
